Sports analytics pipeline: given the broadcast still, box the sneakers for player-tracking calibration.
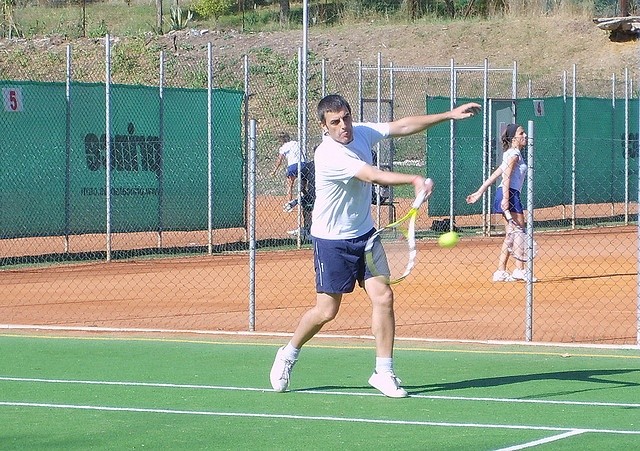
[283,199,297,213]
[368,368,409,397]
[512,269,537,282]
[492,270,517,282]
[269,345,299,392]
[286,229,299,236]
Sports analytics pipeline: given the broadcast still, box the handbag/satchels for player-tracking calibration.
[432,218,463,233]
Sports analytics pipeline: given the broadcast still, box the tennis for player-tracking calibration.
[438,232,459,247]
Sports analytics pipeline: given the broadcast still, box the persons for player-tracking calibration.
[466,124,538,283]
[270,96,483,399]
[271,134,307,203]
[371,150,389,203]
[283,146,324,237]
